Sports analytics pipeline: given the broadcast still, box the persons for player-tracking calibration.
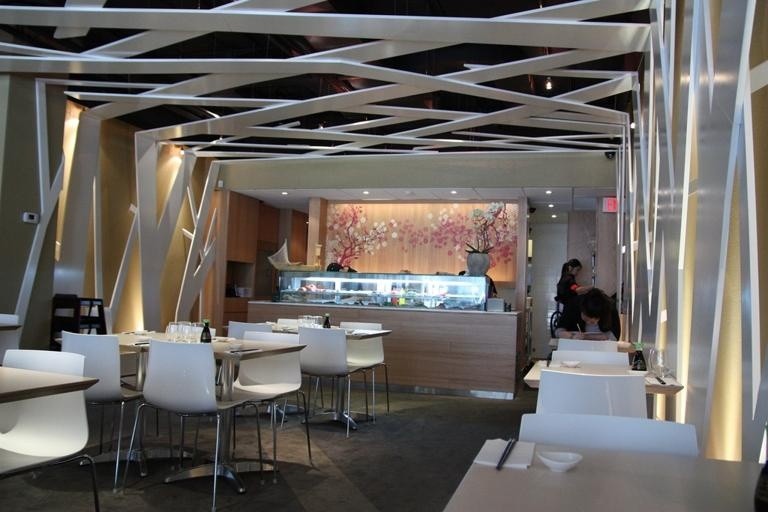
[555,288,621,341]
[554,258,594,309]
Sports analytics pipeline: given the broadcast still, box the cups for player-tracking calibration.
[209,328,216,340]
[167,320,204,344]
[505,302,511,312]
[296,313,322,329]
[526,297,533,307]
[237,287,244,298]
[271,293,280,302]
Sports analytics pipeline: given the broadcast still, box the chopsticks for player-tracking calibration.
[229,348,259,353]
[495,436,517,470]
[655,374,666,385]
[135,342,151,345]
[546,357,551,367]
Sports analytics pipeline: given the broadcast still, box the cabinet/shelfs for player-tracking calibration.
[194,190,308,338]
[276,271,500,311]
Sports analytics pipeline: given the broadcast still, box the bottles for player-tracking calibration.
[399,287,405,306]
[200,319,212,344]
[323,313,331,329]
[391,287,397,305]
[631,350,647,371]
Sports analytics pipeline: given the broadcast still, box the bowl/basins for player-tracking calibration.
[563,361,581,368]
[535,449,583,473]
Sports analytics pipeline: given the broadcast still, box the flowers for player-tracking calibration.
[327,202,401,267]
[403,202,517,268]
[464,203,505,256]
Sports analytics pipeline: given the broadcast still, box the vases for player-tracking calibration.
[468,252,490,276]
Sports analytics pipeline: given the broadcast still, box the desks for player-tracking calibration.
[443,438,766,511]
[523,359,685,396]
[53,337,308,493]
[0,365,100,401]
[220,324,393,425]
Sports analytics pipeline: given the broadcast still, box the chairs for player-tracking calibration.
[231,331,313,484]
[59,329,152,494]
[546,323,633,367]
[121,338,263,512]
[538,370,647,418]
[518,412,700,459]
[280,327,369,437]
[1,348,102,512]
[338,321,391,424]
[135,316,315,468]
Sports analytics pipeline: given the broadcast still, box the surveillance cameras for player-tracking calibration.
[605,153,615,159]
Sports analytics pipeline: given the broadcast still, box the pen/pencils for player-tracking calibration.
[576,323,582,335]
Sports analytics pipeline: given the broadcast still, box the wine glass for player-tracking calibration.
[647,344,666,383]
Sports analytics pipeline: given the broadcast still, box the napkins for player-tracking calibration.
[474,437,536,469]
